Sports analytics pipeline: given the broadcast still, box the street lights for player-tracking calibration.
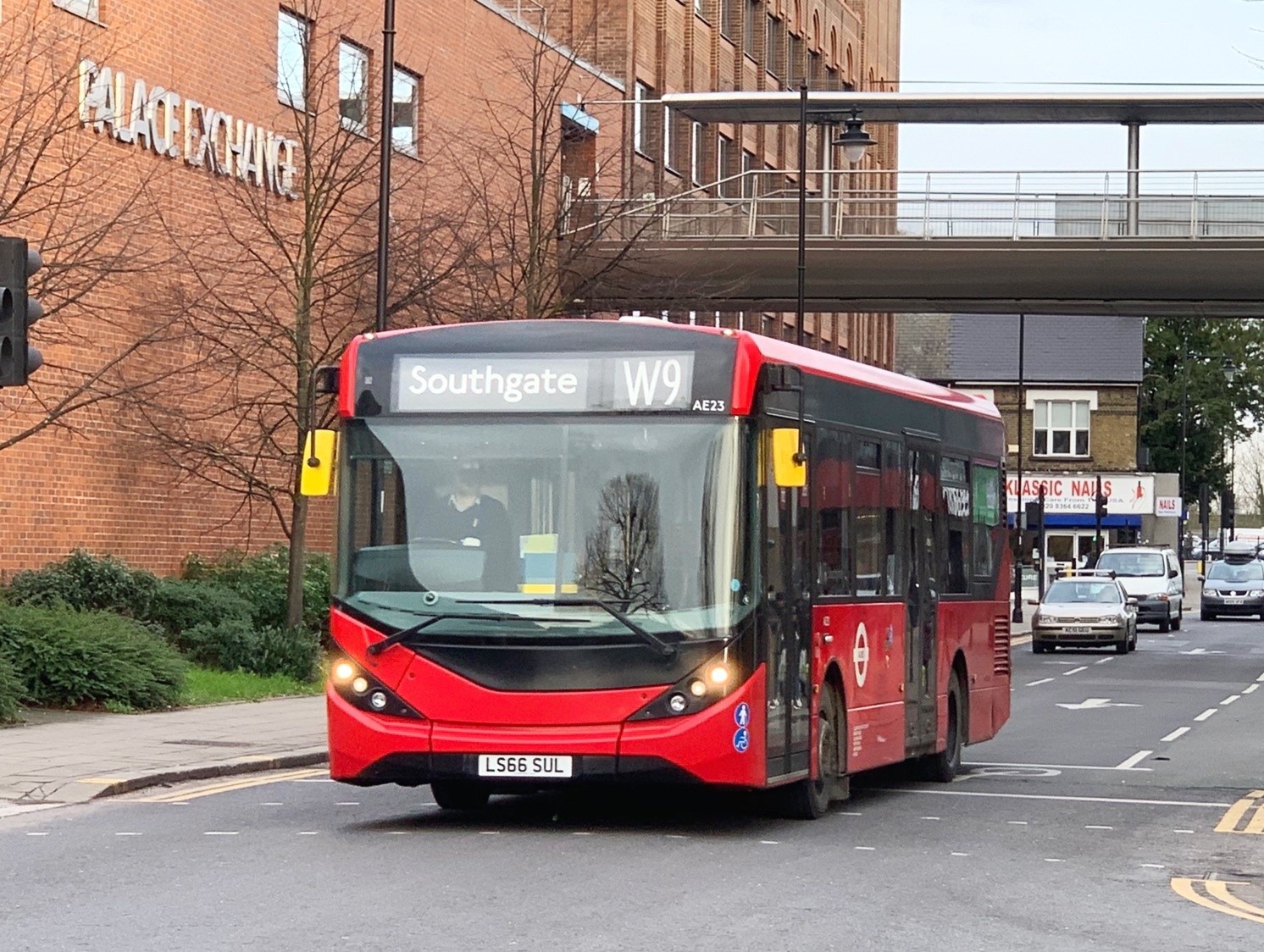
[1178,337,1237,595]
[796,84,878,346]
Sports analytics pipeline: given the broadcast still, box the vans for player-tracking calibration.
[1094,542,1182,632]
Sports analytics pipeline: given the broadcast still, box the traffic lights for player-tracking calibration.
[1199,495,1212,524]
[1096,494,1108,519]
[1,236,45,387]
[1221,490,1234,528]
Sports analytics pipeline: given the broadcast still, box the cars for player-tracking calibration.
[1032,569,1139,654]
[1197,558,1264,621]
[1180,533,1264,559]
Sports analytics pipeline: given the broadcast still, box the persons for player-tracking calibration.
[1184,531,1193,560]
[762,579,810,709]
[415,454,519,593]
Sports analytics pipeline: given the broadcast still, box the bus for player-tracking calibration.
[300,315,1011,818]
